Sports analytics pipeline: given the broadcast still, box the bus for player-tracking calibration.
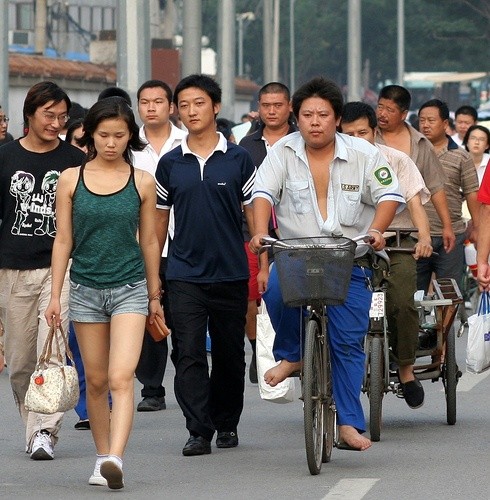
[404,71,490,119]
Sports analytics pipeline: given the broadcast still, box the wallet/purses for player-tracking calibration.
[146,313,170,342]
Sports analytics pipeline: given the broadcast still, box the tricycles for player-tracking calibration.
[360,228,464,441]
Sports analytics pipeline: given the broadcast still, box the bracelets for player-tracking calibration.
[366,229,382,234]
[149,297,161,301]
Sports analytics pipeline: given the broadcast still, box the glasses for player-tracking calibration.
[468,136,487,142]
[38,111,69,123]
[1,117,10,124]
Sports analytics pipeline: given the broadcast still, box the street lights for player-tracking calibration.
[236,12,255,77]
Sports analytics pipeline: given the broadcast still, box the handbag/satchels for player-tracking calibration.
[24,363,80,414]
[256,295,302,403]
[466,290,490,375]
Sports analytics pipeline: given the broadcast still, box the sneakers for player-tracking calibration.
[250,354,258,383]
[100,459,124,489]
[75,419,89,430]
[397,368,426,409]
[88,457,108,485]
[30,432,54,460]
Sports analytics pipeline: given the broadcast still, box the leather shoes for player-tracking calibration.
[183,435,211,455]
[137,396,166,411]
[216,432,238,448]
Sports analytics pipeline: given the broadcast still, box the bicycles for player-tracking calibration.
[257,236,374,475]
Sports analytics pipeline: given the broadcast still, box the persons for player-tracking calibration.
[58,102,84,141]
[0,322,7,373]
[64,116,94,159]
[373,85,456,254]
[445,117,456,137]
[0,81,87,459]
[155,76,269,457]
[44,98,166,489]
[0,106,14,147]
[240,200,262,383]
[476,157,490,292]
[126,81,189,411]
[450,106,478,149]
[460,126,490,227]
[97,88,132,106]
[239,83,301,275]
[170,108,188,134]
[67,117,113,429]
[416,100,480,310]
[408,115,419,131]
[334,102,433,410]
[249,79,406,451]
[240,111,259,122]
[215,118,235,143]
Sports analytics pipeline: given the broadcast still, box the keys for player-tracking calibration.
[356,259,373,292]
